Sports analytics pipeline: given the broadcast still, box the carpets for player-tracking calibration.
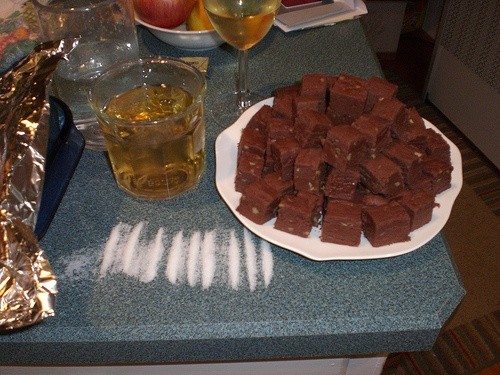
[369,107,500,375]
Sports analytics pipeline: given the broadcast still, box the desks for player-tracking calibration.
[0,16,462,375]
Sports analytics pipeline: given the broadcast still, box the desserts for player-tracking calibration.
[235,71,453,246]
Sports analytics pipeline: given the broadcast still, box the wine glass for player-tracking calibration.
[203,0,281,128]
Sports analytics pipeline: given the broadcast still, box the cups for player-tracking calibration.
[88,57,205,199]
[32,0,140,151]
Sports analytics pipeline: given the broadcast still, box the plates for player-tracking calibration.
[215,96,463,260]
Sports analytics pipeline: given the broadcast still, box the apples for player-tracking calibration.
[132,0,216,31]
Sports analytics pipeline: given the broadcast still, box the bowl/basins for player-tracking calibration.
[134,17,229,51]
